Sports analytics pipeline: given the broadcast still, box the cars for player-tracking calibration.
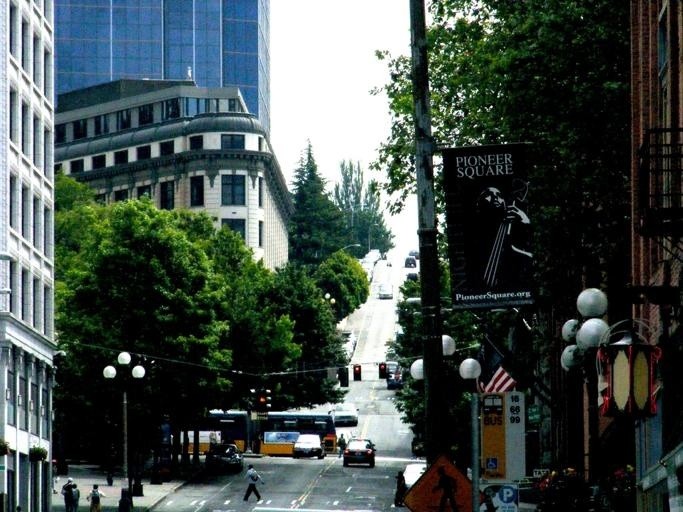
[397,460,427,487]
[291,434,326,460]
[359,248,420,299]
[394,462,432,505]
[203,442,243,471]
[385,361,404,388]
[341,436,376,466]
[326,403,358,426]
[52,450,70,475]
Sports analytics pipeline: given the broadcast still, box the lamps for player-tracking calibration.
[594,311,662,423]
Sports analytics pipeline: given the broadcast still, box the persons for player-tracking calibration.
[394,479,408,507]
[243,464,265,501]
[459,186,533,294]
[71,482,79,512]
[62,476,77,512]
[337,433,346,458]
[86,484,106,512]
[395,471,405,489]
[209,431,217,451]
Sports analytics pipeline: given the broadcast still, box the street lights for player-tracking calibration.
[102,352,147,510]
[560,288,608,511]
[410,334,482,467]
[338,200,378,258]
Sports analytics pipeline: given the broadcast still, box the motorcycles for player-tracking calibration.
[517,466,635,510]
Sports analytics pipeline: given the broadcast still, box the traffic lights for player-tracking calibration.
[336,367,346,380]
[353,364,361,381]
[377,362,387,379]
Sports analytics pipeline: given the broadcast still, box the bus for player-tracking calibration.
[169,407,337,457]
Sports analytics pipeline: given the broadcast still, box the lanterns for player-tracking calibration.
[592,335,664,421]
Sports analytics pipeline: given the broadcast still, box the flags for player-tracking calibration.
[481,364,517,393]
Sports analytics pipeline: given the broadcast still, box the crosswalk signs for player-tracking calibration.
[403,455,487,511]
